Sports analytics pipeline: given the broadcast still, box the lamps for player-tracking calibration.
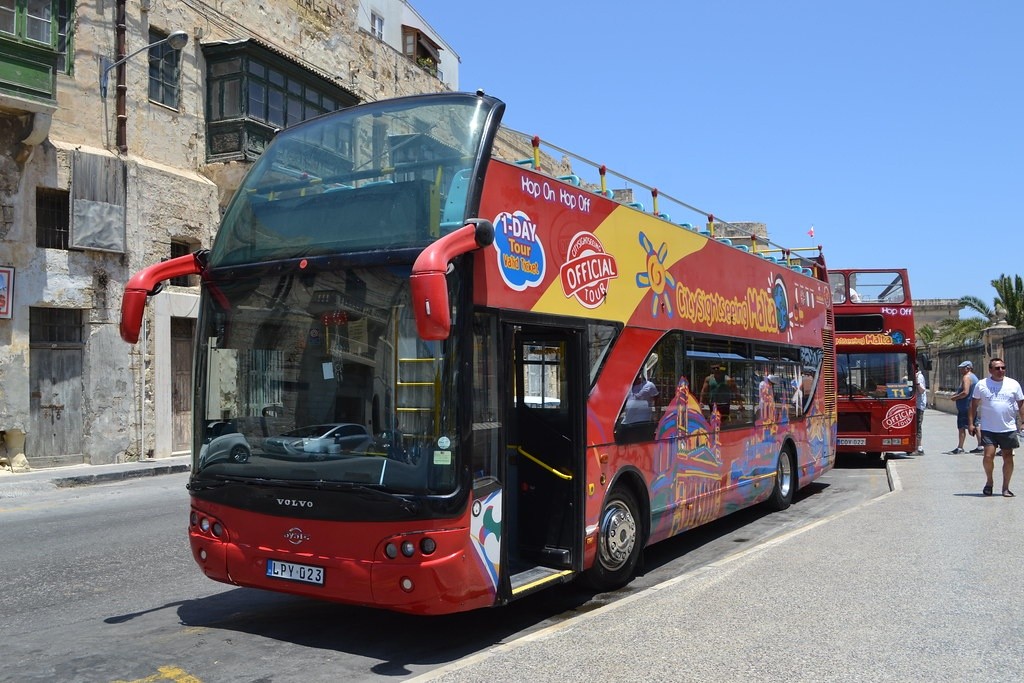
[100,31,188,98]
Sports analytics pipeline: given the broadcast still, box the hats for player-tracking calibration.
[957,361,973,368]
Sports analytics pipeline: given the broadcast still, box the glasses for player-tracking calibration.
[989,366,1006,370]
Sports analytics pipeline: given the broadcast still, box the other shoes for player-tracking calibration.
[1003,489,1014,497]
[983,486,993,496]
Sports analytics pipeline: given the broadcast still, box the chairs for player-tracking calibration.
[324,158,813,277]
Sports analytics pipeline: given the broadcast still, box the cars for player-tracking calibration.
[198,421,253,473]
[259,423,377,458]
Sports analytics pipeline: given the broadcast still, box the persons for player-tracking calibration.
[969,358,1024,497]
[621,372,661,444]
[948,361,1023,457]
[902,361,927,456]
[699,361,741,426]
[836,283,861,302]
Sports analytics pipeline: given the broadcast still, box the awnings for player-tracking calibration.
[687,351,769,364]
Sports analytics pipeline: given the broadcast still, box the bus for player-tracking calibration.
[828,268,932,454]
[117,87,840,615]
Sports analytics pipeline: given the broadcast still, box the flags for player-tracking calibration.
[807,225,814,238]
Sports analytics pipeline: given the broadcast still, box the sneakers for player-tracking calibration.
[906,452,915,455]
[997,450,1016,456]
[917,449,924,455]
[949,447,964,455]
[970,448,984,454]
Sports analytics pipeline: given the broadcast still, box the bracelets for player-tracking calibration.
[1022,422,1024,424]
